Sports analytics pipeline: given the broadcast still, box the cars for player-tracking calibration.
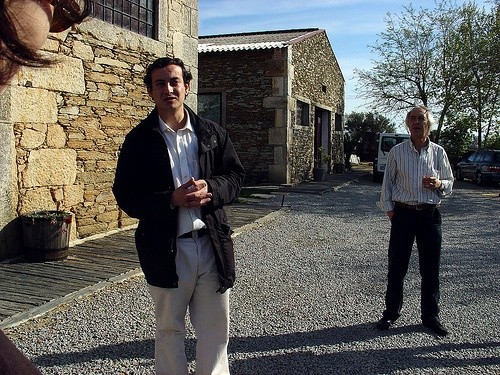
[455,149,500,188]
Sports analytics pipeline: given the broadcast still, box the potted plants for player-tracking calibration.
[313,146,330,182]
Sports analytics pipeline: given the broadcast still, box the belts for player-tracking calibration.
[178,228,208,238]
[395,202,438,210]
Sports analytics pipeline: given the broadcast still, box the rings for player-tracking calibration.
[430,183,433,186]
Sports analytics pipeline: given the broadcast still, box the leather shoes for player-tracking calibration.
[377,317,393,330]
[423,321,449,336]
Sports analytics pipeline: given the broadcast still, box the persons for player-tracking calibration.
[375,107,454,334]
[111,57,244,375]
[0,0,91,79]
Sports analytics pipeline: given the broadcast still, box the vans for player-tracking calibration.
[372,133,412,182]
